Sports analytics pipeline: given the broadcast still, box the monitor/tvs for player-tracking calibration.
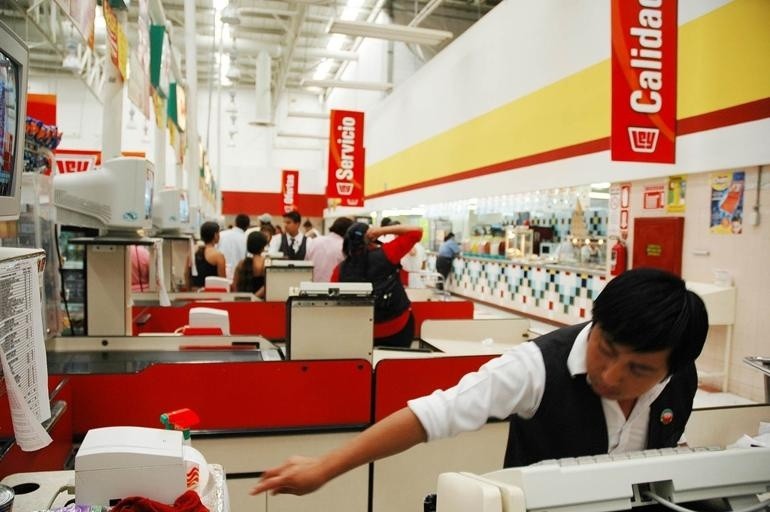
[0,19,30,221]
[191,206,204,240]
[152,189,191,234]
[53,156,156,238]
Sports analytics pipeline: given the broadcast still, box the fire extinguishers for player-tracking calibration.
[610,235,629,276]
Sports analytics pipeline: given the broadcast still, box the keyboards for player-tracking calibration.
[478,446,770,512]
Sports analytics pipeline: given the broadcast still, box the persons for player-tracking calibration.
[185,210,424,348]
[131,244,151,294]
[581,238,602,265]
[249,267,711,497]
[436,232,460,290]
